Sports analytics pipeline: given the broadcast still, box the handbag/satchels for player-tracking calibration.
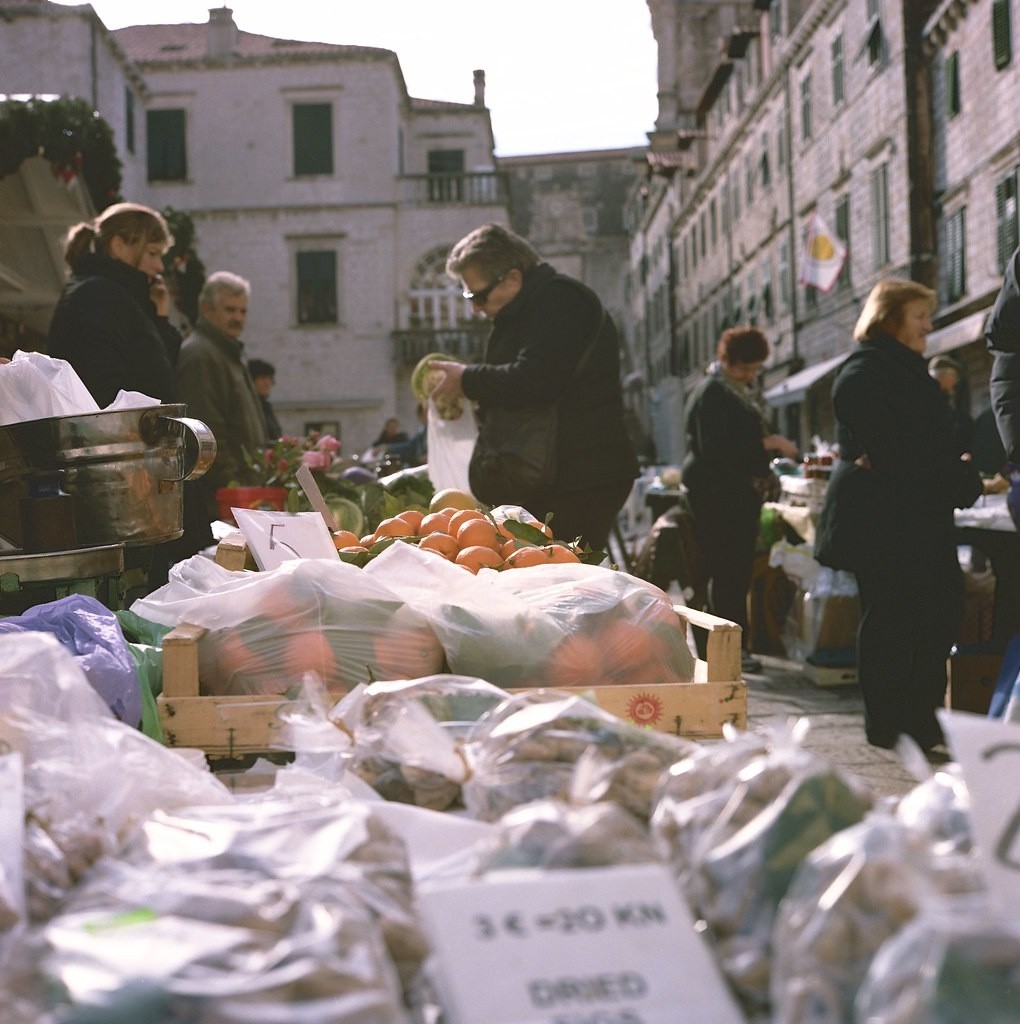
[469,404,566,507]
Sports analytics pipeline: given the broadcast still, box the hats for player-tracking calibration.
[928,355,960,369]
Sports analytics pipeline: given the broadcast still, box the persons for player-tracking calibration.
[370,402,428,468]
[423,221,646,562]
[928,352,962,406]
[174,271,266,549]
[631,491,705,660]
[47,202,183,588]
[679,329,799,674]
[244,359,283,452]
[813,278,983,765]
[983,239,1020,530]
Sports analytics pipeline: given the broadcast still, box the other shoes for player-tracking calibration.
[741,654,760,672]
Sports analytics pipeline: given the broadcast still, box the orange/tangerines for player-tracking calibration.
[208,507,697,691]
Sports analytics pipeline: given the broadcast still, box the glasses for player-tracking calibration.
[462,277,502,306]
